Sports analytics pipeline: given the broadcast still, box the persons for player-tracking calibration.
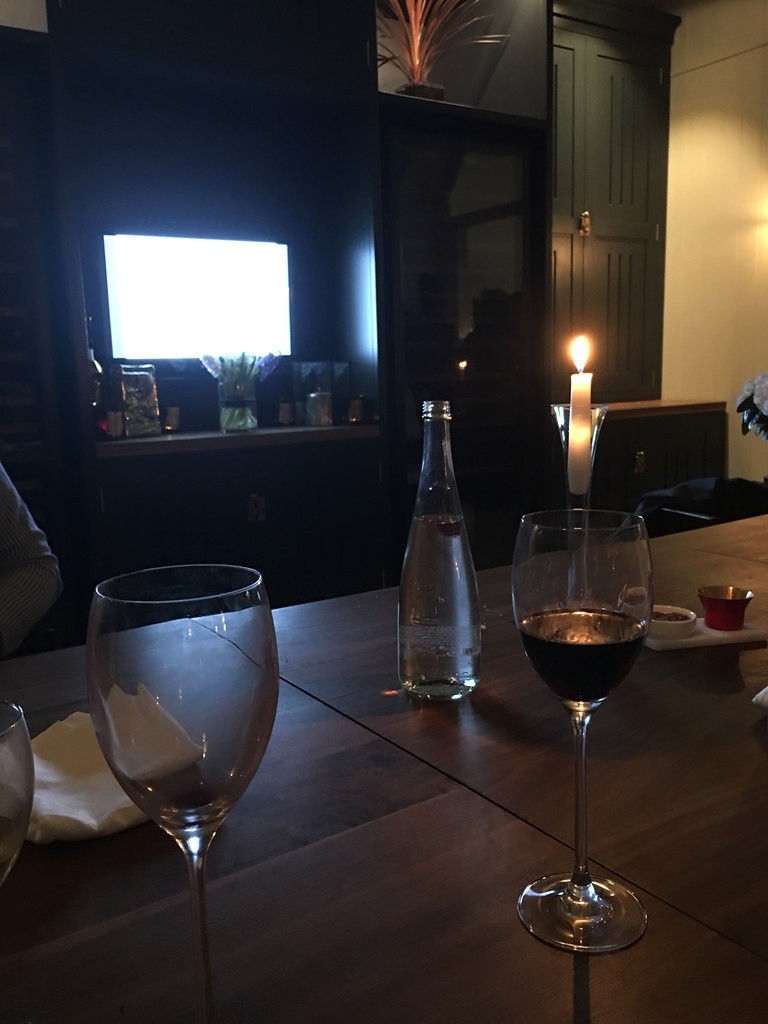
[0,462,62,659]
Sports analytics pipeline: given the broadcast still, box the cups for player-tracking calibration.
[348,394,363,425]
[0,699,34,889]
[216,377,258,433]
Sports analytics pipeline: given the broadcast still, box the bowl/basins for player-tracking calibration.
[645,604,697,639]
[617,582,647,616]
[695,585,753,630]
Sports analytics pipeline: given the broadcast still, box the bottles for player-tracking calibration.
[396,400,483,703]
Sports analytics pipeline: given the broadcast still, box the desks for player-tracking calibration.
[195,514,768,963]
[0,627,768,1024]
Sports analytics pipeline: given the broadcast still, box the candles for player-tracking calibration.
[565,336,594,495]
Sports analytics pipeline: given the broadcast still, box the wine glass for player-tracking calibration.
[510,506,648,954]
[84,564,279,1024]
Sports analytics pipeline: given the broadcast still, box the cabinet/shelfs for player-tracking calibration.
[551,0,682,405]
[553,400,729,529]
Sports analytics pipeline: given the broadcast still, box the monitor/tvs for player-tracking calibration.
[103,234,292,360]
